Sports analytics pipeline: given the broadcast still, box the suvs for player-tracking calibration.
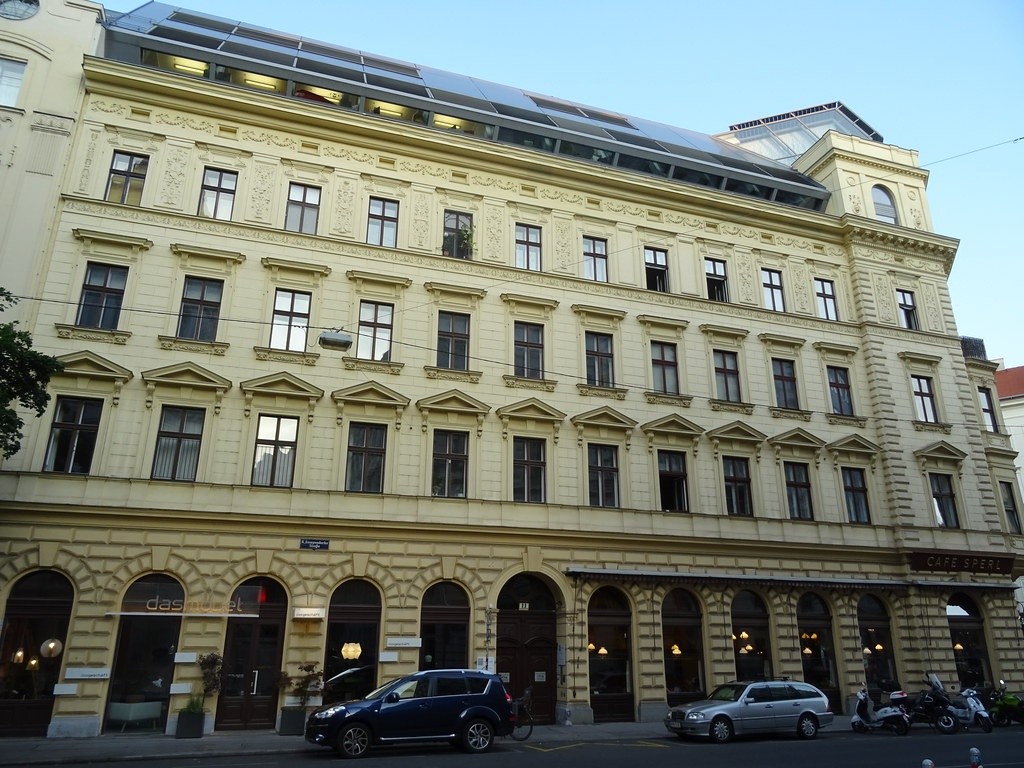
[304,667,516,760]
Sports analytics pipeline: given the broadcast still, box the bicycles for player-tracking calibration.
[508,698,533,742]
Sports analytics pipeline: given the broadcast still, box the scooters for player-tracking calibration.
[987,679,1024,728]
[850,681,910,737]
[938,680,993,734]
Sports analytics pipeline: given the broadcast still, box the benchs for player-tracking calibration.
[107,700,162,733]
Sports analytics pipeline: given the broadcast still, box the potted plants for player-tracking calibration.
[177,653,231,738]
[279,660,326,735]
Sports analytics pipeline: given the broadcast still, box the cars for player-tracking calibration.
[663,675,835,744]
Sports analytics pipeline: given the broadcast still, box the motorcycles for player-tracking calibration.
[900,670,959,735]
